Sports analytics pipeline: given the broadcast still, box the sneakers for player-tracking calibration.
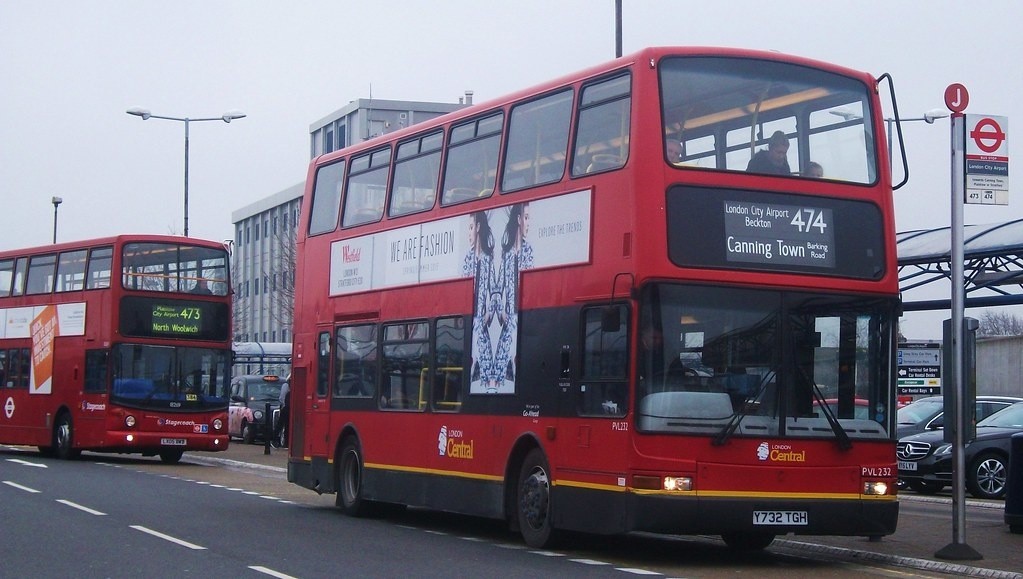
[270,440,279,450]
[281,444,289,450]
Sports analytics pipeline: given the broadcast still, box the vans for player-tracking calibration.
[226,376,287,446]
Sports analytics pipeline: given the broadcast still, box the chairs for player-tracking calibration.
[352,151,619,225]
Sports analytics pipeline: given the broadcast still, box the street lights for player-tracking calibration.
[51,196,65,245]
[125,109,248,236]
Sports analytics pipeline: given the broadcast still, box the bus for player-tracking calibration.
[0,233,237,462]
[284,46,907,554]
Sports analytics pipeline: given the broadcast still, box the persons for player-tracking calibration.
[805,161,823,177]
[638,325,684,376]
[189,280,212,294]
[462,202,535,391]
[747,130,790,174]
[270,378,290,449]
[668,138,682,163]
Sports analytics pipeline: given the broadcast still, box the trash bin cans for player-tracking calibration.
[1004,432,1023,534]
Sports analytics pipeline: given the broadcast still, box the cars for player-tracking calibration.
[809,394,1023,501]
